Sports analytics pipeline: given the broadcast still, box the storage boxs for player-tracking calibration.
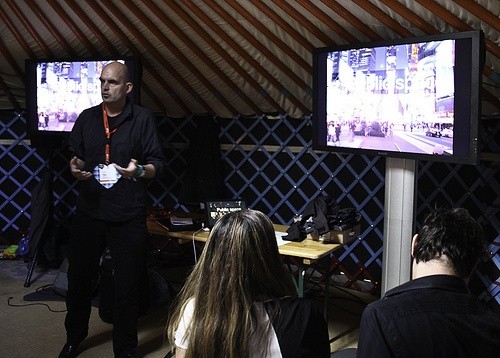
[309,225,361,245]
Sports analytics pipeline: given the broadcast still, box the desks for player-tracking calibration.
[148,222,345,321]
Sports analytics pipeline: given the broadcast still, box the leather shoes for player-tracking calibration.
[114,346,136,358]
[59,334,85,357]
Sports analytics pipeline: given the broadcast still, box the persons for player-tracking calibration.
[357,208,500,358]
[58,61,164,358]
[327,115,453,142]
[164,210,331,358]
[39,107,79,134]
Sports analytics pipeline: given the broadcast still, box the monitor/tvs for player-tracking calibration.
[24,54,141,139]
[312,29,486,166]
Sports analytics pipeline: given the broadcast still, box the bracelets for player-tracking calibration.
[134,165,146,179]
